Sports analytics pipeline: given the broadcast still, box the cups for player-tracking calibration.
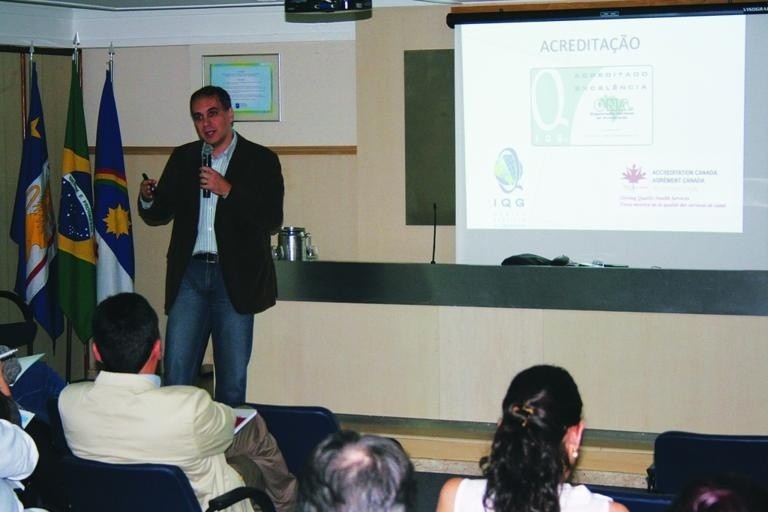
[307,246,319,261]
[271,245,284,259]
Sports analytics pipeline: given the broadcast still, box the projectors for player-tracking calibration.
[284,0,372,23]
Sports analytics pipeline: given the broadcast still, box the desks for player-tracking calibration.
[211,261,768,474]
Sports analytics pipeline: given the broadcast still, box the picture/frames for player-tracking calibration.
[200,53,282,123]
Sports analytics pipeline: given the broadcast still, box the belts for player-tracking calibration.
[195,252,219,263]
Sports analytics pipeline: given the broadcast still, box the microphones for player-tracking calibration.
[431,203,436,263]
[202,144,214,198]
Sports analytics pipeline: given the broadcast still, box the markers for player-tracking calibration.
[142,172,154,191]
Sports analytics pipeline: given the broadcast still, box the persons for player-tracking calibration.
[301,429,417,512]
[435,365,631,512]
[58,292,301,512]
[136,85,285,408]
[0,358,54,512]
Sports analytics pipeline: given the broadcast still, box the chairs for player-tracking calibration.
[224,402,337,464]
[58,456,275,511]
[646,430,768,511]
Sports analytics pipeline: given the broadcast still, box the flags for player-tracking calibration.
[95,69,134,303]
[10,62,65,356]
[54,55,95,345]
